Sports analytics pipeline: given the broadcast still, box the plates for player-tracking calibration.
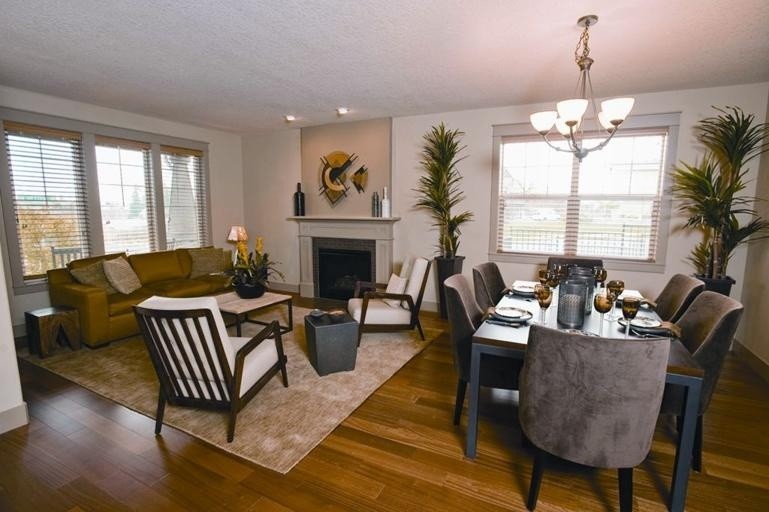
[509,282,536,296]
[493,307,534,322]
[618,316,668,334]
[617,296,647,307]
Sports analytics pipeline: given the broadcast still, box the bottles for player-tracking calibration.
[372,191,380,217]
[293,183,306,217]
[309,309,324,320]
[381,186,392,218]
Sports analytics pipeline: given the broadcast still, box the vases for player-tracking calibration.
[234,286,265,300]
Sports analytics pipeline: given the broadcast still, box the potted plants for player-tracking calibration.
[410,121,476,319]
[664,103,769,295]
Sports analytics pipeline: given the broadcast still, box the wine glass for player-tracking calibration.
[594,295,612,339]
[535,284,554,325]
[596,269,607,294]
[539,268,558,288]
[606,280,624,320]
[622,297,640,341]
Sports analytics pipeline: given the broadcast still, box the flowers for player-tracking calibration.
[224,235,286,289]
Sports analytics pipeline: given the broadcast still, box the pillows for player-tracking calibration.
[383,273,408,309]
[140,298,236,381]
[400,257,428,310]
[65,245,232,294]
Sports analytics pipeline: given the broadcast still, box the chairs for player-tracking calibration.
[348,255,431,347]
[132,296,288,442]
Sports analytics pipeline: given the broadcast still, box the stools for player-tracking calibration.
[304,312,359,377]
[24,306,80,359]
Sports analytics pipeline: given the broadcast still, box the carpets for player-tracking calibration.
[15,302,444,475]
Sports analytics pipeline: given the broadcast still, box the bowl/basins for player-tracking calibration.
[327,308,346,321]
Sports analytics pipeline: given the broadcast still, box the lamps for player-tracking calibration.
[529,15,635,164]
[227,225,248,267]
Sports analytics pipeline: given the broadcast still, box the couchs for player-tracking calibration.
[47,245,236,349]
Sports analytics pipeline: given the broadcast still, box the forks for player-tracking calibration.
[632,330,674,340]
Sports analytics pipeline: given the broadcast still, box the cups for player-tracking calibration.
[571,267,595,315]
[558,279,586,328]
